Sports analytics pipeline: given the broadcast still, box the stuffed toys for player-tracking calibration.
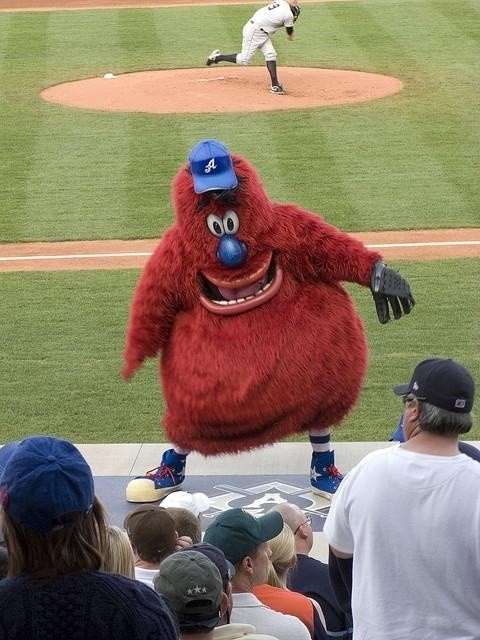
[116,138,415,506]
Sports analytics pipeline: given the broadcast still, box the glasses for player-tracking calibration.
[401,396,426,403]
[294,514,314,535]
[178,610,221,627]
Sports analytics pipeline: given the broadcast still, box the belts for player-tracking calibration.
[249,20,269,35]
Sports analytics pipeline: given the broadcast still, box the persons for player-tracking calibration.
[205,1,301,96]
[322,357,480,640]
[0,435,180,637]
[96,501,352,639]
[388,414,479,461]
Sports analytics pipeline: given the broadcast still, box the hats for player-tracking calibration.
[287,0,297,6]
[1,437,95,532]
[124,505,177,562]
[204,508,284,564]
[392,359,476,414]
[179,543,236,582]
[154,550,223,609]
[159,491,210,518]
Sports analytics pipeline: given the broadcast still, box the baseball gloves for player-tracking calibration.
[372,260,416,324]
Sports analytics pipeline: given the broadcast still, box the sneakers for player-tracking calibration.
[270,84,284,95]
[206,49,221,66]
